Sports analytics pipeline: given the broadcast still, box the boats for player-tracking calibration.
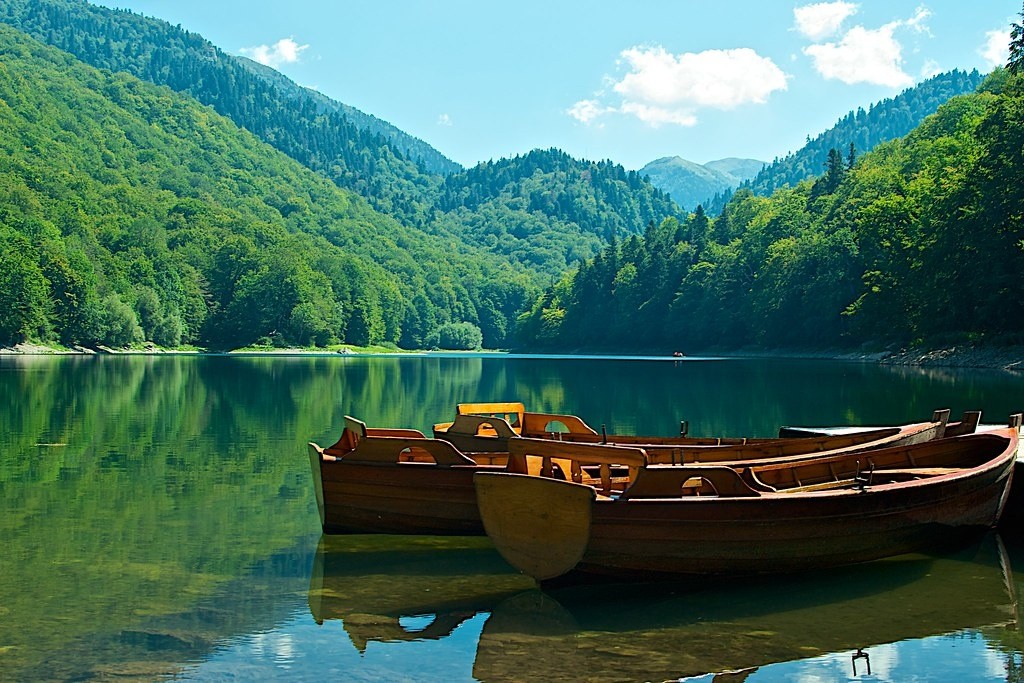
[306,401,1022,587]
